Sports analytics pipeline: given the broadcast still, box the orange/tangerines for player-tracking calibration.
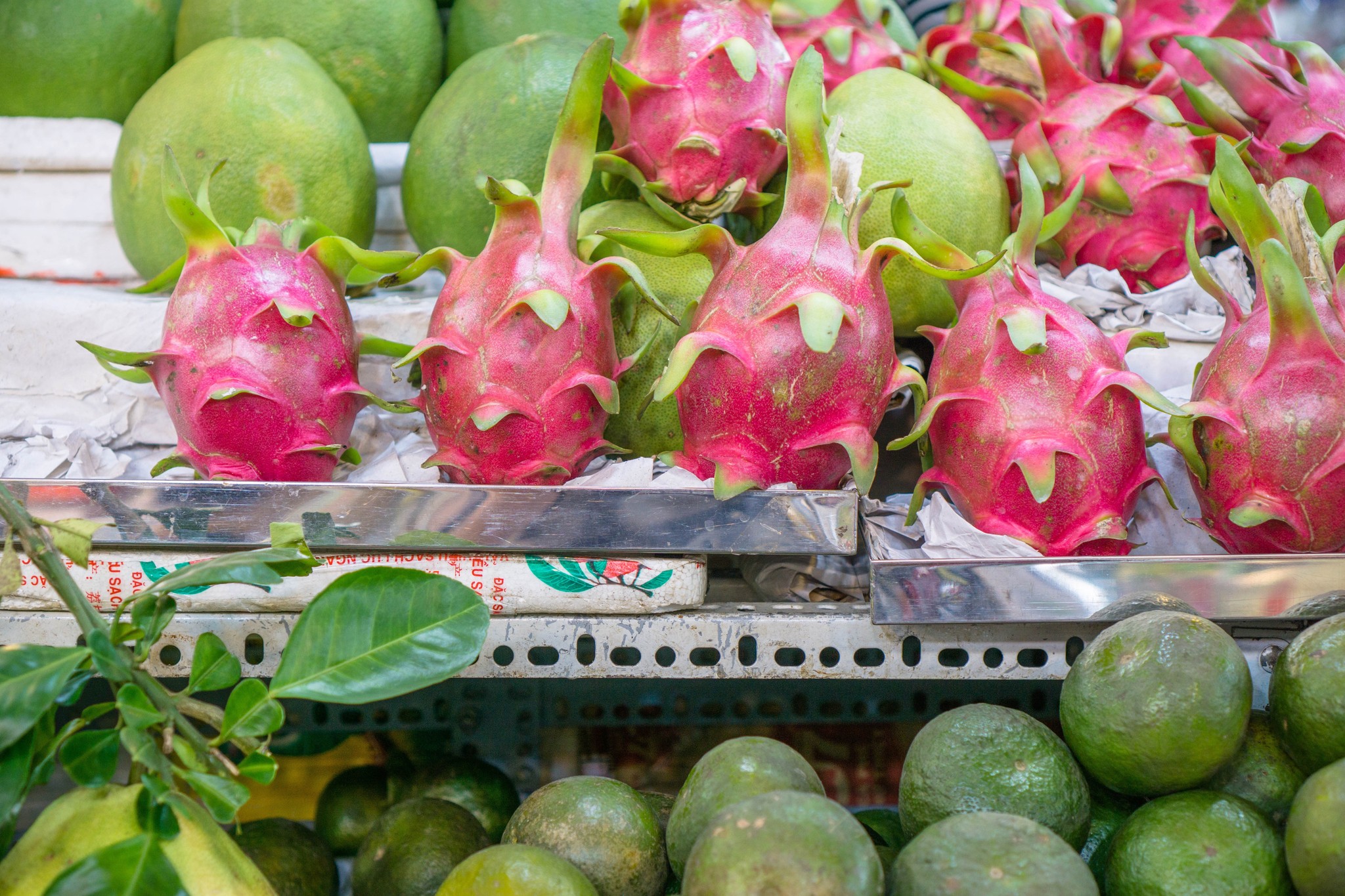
[225,609,1345,896]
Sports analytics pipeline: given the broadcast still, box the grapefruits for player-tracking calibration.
[0,783,278,896]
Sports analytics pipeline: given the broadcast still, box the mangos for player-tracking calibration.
[0,0,1014,456]
